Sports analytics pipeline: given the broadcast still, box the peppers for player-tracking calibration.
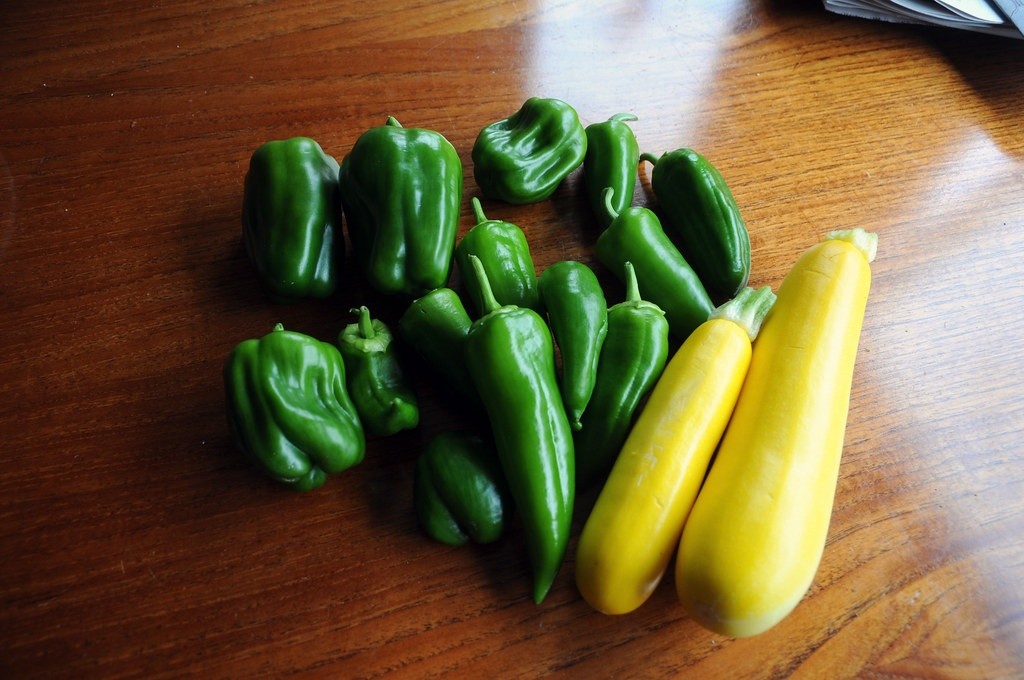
[225,94,752,607]
